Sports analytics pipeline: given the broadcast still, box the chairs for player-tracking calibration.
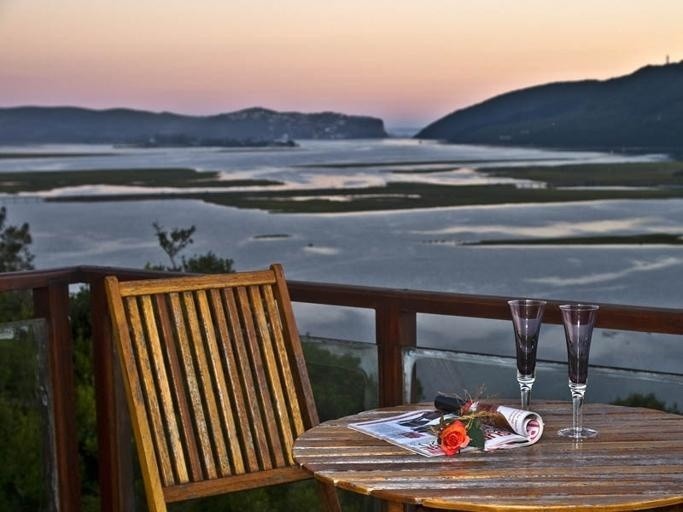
[105,263,344,511]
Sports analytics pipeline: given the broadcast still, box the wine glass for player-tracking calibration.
[558,299,600,440]
[507,300,548,412]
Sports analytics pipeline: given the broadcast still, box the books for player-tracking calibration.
[348,392,544,458]
